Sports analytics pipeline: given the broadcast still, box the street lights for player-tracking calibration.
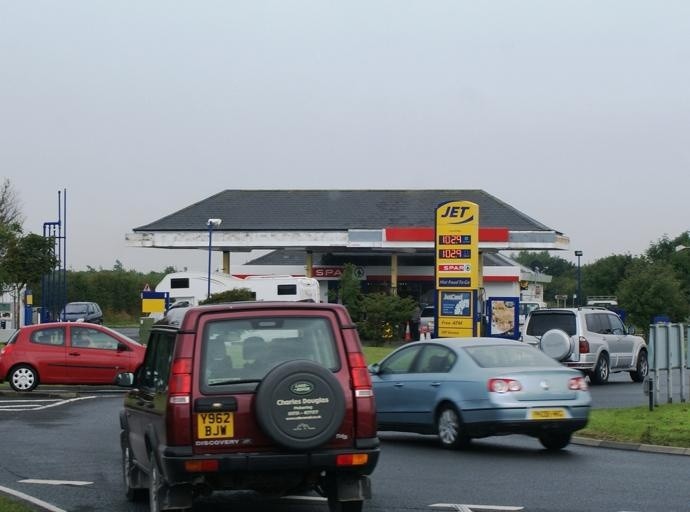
[204,214,225,307]
[574,248,585,306]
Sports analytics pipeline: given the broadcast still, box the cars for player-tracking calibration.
[366,334,596,452]
[415,304,434,330]
[55,301,104,329]
[0,320,148,392]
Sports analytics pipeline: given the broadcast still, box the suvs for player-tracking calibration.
[117,297,383,511]
[520,305,651,385]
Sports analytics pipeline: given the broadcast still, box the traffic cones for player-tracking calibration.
[403,319,413,343]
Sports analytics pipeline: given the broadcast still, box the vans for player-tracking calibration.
[517,300,547,335]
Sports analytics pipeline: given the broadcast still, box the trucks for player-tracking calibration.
[149,271,322,345]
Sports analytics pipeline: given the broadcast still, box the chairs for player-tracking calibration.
[243,337,265,366]
[207,338,231,371]
[422,356,450,372]
